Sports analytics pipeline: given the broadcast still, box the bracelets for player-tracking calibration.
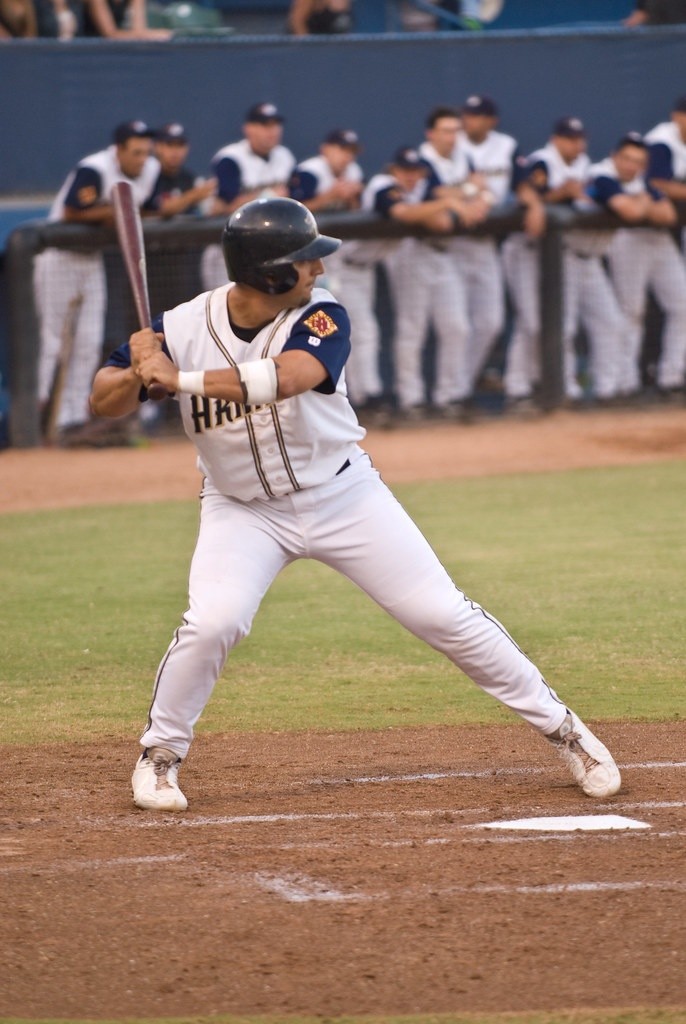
[458,182,477,199]
[177,370,205,395]
[445,210,463,231]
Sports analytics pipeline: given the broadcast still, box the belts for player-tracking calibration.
[336,459,350,475]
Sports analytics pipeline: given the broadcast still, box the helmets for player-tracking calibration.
[222,197,342,294]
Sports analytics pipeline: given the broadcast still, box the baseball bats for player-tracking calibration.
[108,180,168,402]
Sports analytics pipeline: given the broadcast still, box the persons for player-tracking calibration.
[0,1,177,44]
[89,200,624,809]
[623,0,686,27]
[33,120,162,443]
[382,92,547,421]
[284,128,384,414]
[198,101,298,293]
[565,129,678,400]
[288,0,352,37]
[151,119,227,325]
[334,144,481,416]
[499,118,595,414]
[402,1,504,32]
[604,94,686,390]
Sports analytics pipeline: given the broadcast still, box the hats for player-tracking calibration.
[153,122,188,143]
[247,101,285,122]
[463,95,495,115]
[327,127,360,148]
[619,133,650,148]
[551,117,588,138]
[115,120,156,142]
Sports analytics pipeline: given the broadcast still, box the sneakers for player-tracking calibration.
[542,707,621,799]
[131,746,188,813]
